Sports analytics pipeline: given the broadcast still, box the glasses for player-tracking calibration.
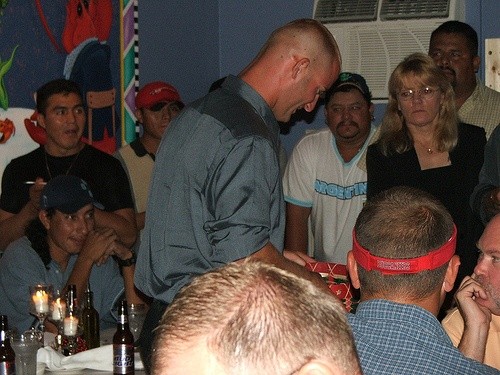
[394,87,442,100]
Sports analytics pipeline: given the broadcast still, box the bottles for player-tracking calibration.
[83,292,100,350]
[61,284,87,356]
[112,301,135,375]
[0,314,16,375]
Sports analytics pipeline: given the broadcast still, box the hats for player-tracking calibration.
[325,72,369,97]
[134,82,186,110]
[39,175,105,214]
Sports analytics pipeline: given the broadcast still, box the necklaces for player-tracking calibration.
[44,141,83,180]
[419,144,436,152]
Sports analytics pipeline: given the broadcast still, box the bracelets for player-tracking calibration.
[118,253,138,267]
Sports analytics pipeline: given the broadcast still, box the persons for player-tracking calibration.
[427,20,500,142]
[133,18,341,375]
[346,187,500,375]
[150,256,362,375]
[366,53,487,322]
[110,81,185,226]
[441,212,500,370]
[281,72,384,264]
[468,122,500,228]
[0,79,138,249]
[0,175,149,331]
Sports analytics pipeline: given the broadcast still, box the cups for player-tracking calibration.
[127,303,146,350]
[9,333,38,375]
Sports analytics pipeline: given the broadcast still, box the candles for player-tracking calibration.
[32,291,49,314]
[52,299,65,320]
[64,315,79,335]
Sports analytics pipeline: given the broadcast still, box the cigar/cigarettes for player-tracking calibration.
[25,182,35,184]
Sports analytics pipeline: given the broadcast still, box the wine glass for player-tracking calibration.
[28,284,54,348]
[47,286,67,351]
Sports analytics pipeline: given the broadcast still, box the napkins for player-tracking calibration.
[36,344,145,371]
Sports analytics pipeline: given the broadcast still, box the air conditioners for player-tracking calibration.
[311,0,466,105]
